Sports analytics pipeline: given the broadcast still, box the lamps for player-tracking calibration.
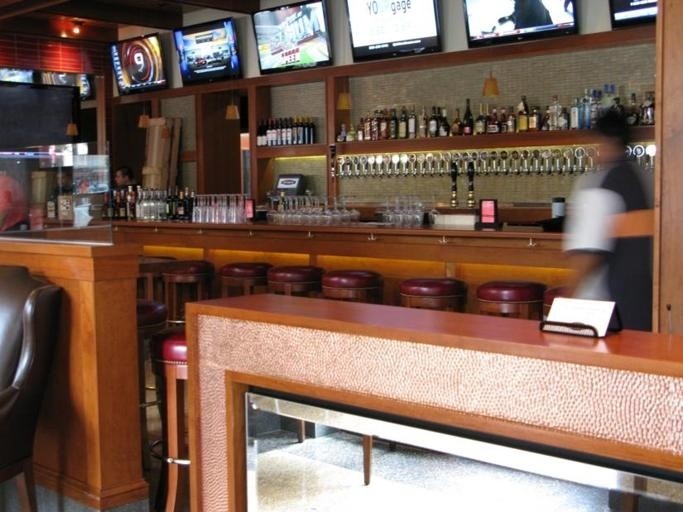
[63,86,80,137]
[336,75,356,111]
[137,98,153,128]
[224,91,241,119]
[479,57,500,98]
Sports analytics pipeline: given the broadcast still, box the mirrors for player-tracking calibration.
[243,387,682,511]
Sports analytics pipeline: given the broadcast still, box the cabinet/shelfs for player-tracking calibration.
[245,24,656,222]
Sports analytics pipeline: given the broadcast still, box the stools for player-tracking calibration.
[133,250,216,511]
[542,284,585,327]
[474,279,547,323]
[320,268,381,302]
[219,261,272,299]
[265,266,325,296]
[294,413,397,486]
[399,277,469,312]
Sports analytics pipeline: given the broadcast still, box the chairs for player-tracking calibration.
[0,262,71,511]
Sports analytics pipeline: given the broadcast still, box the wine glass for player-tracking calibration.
[192,191,247,224]
[265,192,359,226]
[380,195,427,228]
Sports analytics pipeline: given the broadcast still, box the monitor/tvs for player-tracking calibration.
[108,32,169,96]
[608,0,658,31]
[0,66,38,85]
[173,16,242,87]
[345,0,442,63]
[38,70,95,100]
[252,0,333,74]
[463,1,577,48]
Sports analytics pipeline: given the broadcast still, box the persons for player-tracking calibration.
[558,111,651,331]
[191,51,195,72]
[564,0,573,14]
[0,165,30,231]
[218,46,224,64]
[499,0,553,30]
[114,165,141,191]
[53,170,73,213]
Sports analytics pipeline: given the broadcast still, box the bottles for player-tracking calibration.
[550,196,566,218]
[99,184,194,223]
[46,194,56,218]
[255,115,316,147]
[337,85,655,142]
